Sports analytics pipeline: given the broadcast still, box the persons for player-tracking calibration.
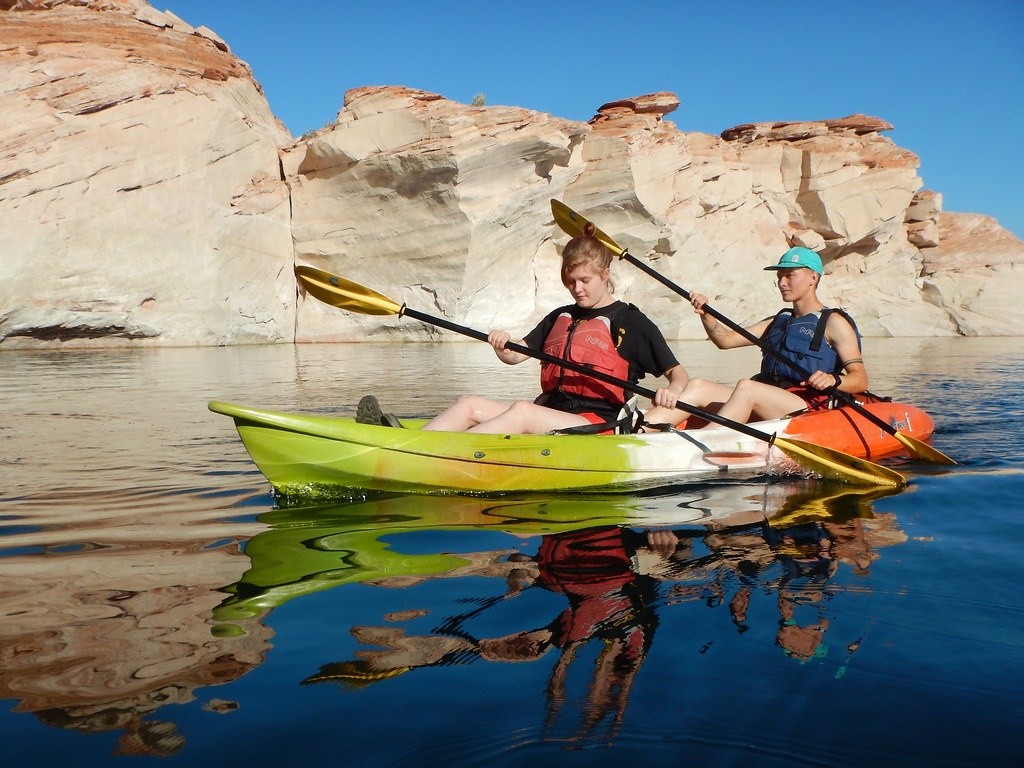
[637,246,869,433]
[355,221,690,436]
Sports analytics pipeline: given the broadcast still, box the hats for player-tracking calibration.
[763,246,823,276]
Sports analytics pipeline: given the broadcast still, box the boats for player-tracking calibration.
[204,397,935,499]
[211,456,926,633]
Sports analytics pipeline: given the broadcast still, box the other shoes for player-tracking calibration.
[380,411,404,429]
[355,394,383,425]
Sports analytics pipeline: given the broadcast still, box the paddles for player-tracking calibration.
[293,264,907,487]
[550,196,960,465]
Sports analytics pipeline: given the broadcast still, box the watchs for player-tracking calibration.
[827,372,842,389]
